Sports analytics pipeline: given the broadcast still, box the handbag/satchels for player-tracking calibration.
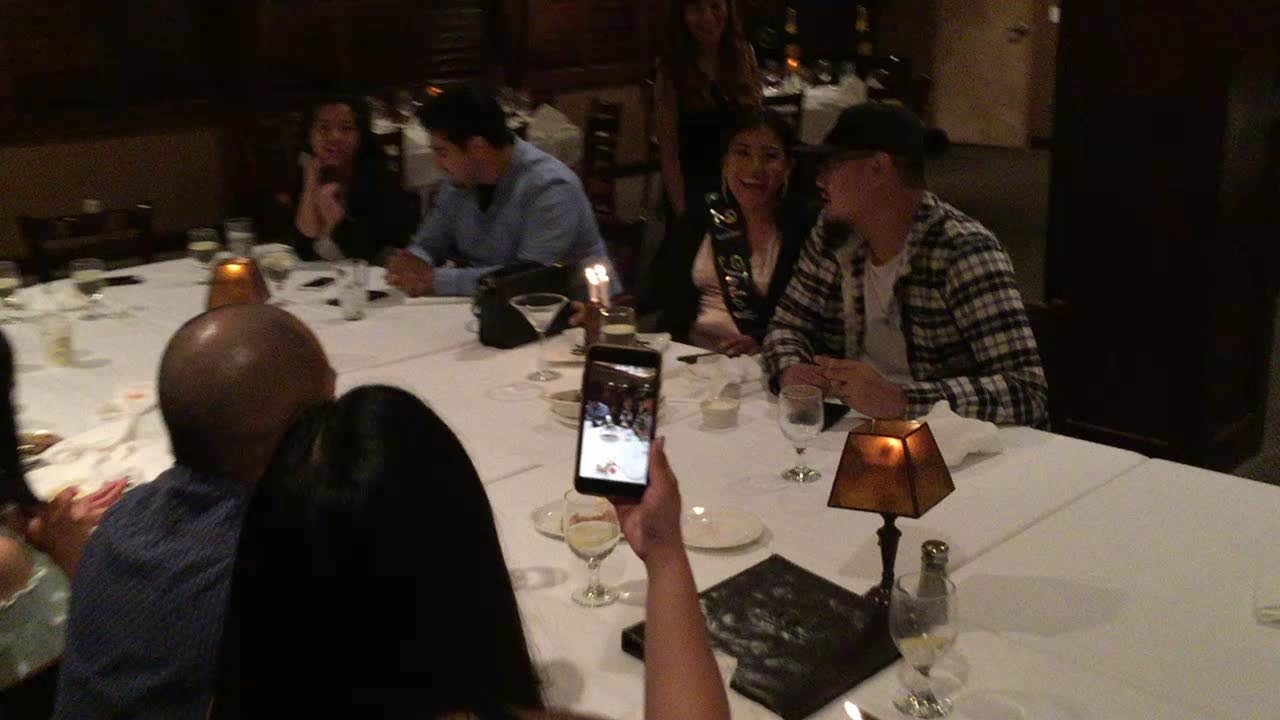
[471,258,590,350]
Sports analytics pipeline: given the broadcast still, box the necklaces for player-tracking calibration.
[866,268,898,323]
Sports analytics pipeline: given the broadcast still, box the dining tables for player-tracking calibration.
[0,239,1280,720]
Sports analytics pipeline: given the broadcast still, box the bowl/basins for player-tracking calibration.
[547,388,581,418]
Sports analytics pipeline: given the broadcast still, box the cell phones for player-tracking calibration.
[790,401,849,430]
[573,342,665,498]
[303,277,334,288]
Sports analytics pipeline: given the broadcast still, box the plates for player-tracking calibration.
[548,411,580,428]
[676,503,762,549]
[540,331,672,364]
[529,500,625,536]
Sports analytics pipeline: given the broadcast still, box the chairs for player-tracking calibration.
[583,98,631,240]
[16,203,153,282]
[760,94,806,132]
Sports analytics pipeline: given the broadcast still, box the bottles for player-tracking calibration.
[917,540,951,627]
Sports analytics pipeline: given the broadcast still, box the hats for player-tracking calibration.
[791,103,924,153]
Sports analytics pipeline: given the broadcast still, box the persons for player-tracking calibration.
[582,378,652,443]
[0,330,128,720]
[759,100,1054,431]
[257,91,396,264]
[55,304,337,720]
[209,370,735,720]
[562,108,812,357]
[382,86,626,311]
[654,0,762,221]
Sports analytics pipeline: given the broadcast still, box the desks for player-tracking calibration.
[393,91,582,212]
[767,63,869,145]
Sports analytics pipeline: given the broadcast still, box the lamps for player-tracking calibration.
[206,257,273,308]
[827,419,957,596]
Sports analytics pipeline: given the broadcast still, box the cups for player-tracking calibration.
[702,396,741,427]
[599,304,635,346]
[185,226,219,265]
[335,258,368,321]
[225,218,253,259]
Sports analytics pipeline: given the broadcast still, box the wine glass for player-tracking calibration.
[0,261,24,325]
[70,258,105,320]
[890,571,959,720]
[510,294,567,380]
[564,490,625,607]
[777,384,825,483]
